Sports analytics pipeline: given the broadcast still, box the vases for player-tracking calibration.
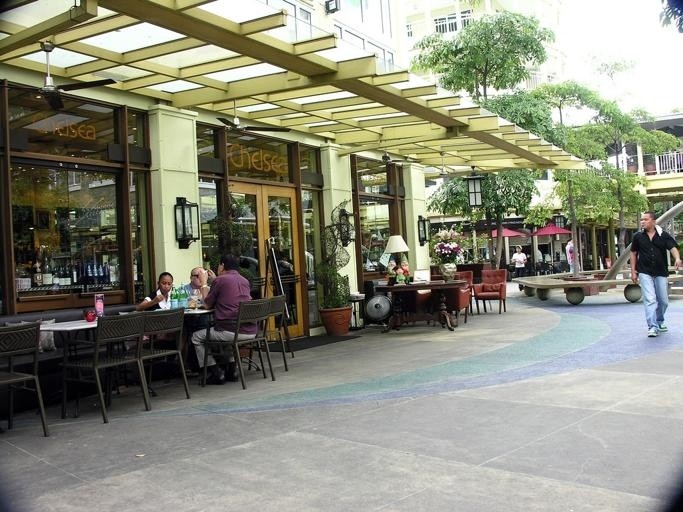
[439,264,457,283]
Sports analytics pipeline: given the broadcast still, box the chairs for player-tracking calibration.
[473,268,507,314]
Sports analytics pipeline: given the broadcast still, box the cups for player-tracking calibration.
[85,310,96,322]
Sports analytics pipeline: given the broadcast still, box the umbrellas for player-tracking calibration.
[527,222,575,274]
[491,227,526,239]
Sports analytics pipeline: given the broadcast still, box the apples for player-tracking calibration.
[83,308,96,322]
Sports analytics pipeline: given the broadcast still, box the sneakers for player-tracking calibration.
[656,323,668,332]
[648,327,659,337]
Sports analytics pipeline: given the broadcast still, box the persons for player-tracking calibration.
[176,266,211,377]
[135,272,199,378]
[629,212,682,337]
[565,237,580,273]
[511,245,528,278]
[191,251,259,385]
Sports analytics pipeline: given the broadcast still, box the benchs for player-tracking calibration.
[387,271,473,323]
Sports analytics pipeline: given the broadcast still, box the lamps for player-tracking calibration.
[554,212,565,229]
[385,234,410,286]
[462,166,486,209]
[417,215,431,246]
[173,196,200,249]
[339,209,356,246]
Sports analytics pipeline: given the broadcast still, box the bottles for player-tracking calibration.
[95,294,104,317]
[16,262,111,288]
[170,283,179,309]
[179,282,189,308]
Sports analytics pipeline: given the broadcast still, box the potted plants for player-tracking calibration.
[207,183,258,358]
[312,198,357,337]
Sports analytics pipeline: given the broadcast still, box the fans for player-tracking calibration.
[216,98,292,135]
[424,151,469,180]
[357,151,423,171]
[0,41,116,110]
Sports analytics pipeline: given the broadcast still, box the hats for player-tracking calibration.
[516,245,522,249]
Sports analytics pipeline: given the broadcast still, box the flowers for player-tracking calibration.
[433,242,463,264]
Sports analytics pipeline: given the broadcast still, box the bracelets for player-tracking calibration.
[201,284,210,292]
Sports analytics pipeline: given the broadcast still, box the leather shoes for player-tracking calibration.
[225,371,239,382]
[200,373,225,385]
